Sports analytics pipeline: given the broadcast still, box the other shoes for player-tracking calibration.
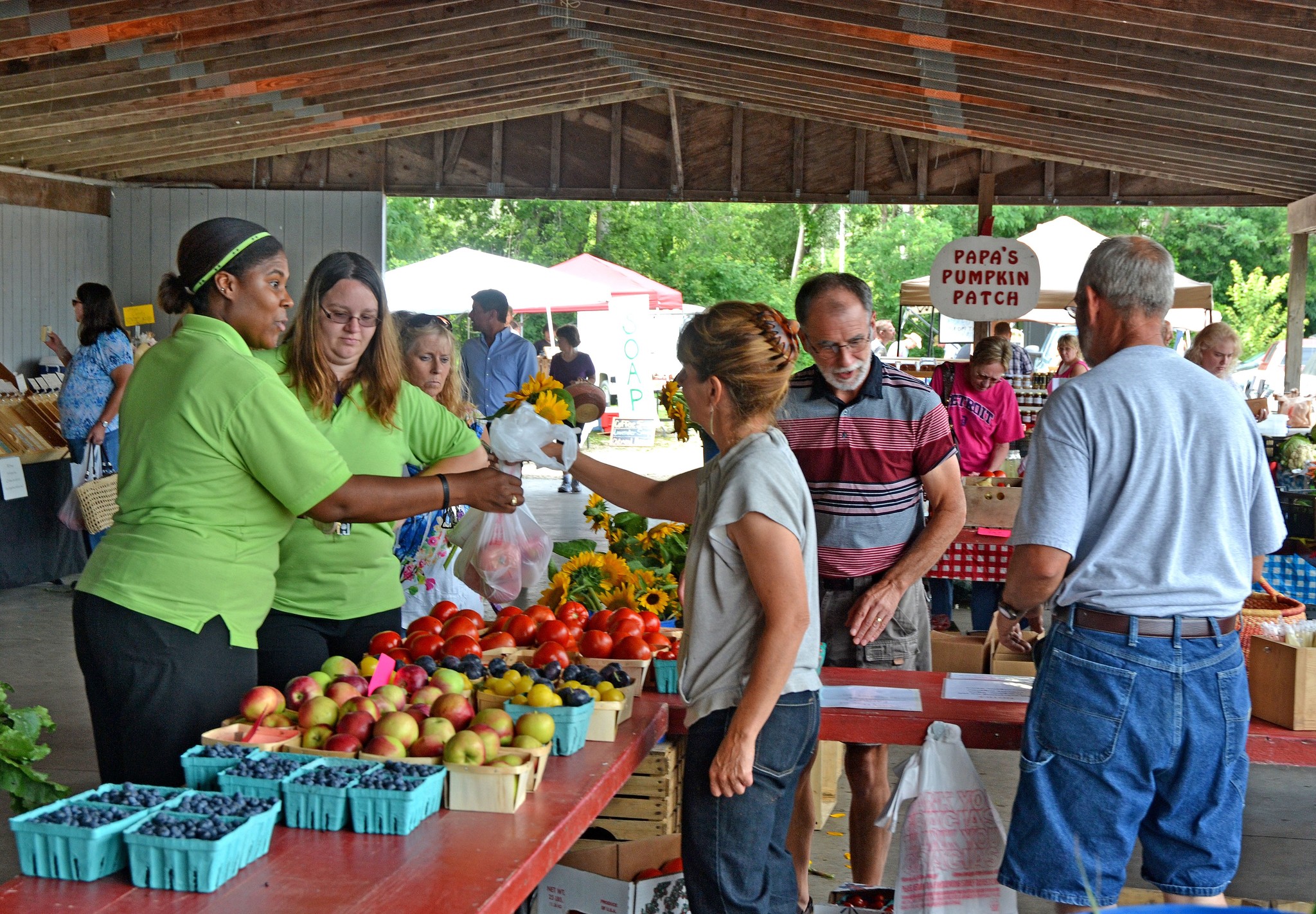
[796,896,814,914]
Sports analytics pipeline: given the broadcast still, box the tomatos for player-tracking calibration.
[978,471,1025,487]
[637,868,664,880]
[661,857,683,875]
[369,600,681,669]
[463,537,545,603]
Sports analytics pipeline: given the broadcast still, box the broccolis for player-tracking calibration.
[1287,445,1316,469]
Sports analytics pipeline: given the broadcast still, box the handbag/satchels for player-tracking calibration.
[57,437,104,531]
[1245,398,1268,416]
[447,406,577,604]
[929,362,962,463]
[76,437,118,535]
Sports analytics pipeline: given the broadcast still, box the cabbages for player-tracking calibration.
[1277,423,1316,466]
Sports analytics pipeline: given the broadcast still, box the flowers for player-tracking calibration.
[536,493,697,627]
[442,373,577,570]
[657,381,704,444]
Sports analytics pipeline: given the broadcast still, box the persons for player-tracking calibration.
[460,289,539,471]
[543,300,819,914]
[871,319,896,357]
[1047,333,1089,399]
[44,282,134,590]
[992,321,1033,375]
[931,334,962,359]
[1183,322,1270,423]
[42,217,1287,914]
[548,324,595,494]
[928,336,1024,631]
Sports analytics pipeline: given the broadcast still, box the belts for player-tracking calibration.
[1053,605,1235,639]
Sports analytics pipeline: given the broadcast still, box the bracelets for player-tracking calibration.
[435,474,450,509]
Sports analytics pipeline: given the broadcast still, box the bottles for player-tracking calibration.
[1000,450,1021,478]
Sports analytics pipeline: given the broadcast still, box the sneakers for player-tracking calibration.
[571,478,582,492]
[558,477,572,493]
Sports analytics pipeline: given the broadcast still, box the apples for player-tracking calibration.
[241,656,554,803]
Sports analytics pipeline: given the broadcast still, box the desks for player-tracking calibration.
[0,458,92,589]
[922,529,1044,635]
[0,700,669,914]
[638,668,1316,782]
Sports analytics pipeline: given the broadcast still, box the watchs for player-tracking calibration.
[98,420,110,428]
[997,601,1026,620]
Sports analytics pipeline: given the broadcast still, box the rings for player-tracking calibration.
[876,618,883,622]
[511,495,517,506]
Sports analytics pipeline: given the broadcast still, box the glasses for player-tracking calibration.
[72,299,82,306]
[972,367,1005,383]
[318,302,383,327]
[1064,296,1078,318]
[400,314,453,343]
[801,321,871,359]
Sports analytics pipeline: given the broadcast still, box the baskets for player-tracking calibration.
[1234,574,1306,679]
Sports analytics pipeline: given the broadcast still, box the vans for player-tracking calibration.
[1231,334,1316,399]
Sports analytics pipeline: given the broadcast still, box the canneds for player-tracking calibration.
[1004,372,1054,424]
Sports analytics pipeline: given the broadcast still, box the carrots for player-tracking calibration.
[1305,461,1316,478]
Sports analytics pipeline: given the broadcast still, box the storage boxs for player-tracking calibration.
[962,476,1025,527]
[538,355,551,377]
[930,627,1045,677]
[10,632,695,914]
[1247,634,1316,733]
[0,362,72,465]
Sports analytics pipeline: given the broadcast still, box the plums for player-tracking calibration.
[394,654,631,707]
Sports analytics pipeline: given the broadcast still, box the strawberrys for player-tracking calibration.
[843,893,894,912]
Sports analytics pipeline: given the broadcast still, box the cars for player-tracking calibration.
[1034,324,1081,374]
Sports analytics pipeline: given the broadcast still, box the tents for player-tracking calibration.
[382,247,682,450]
